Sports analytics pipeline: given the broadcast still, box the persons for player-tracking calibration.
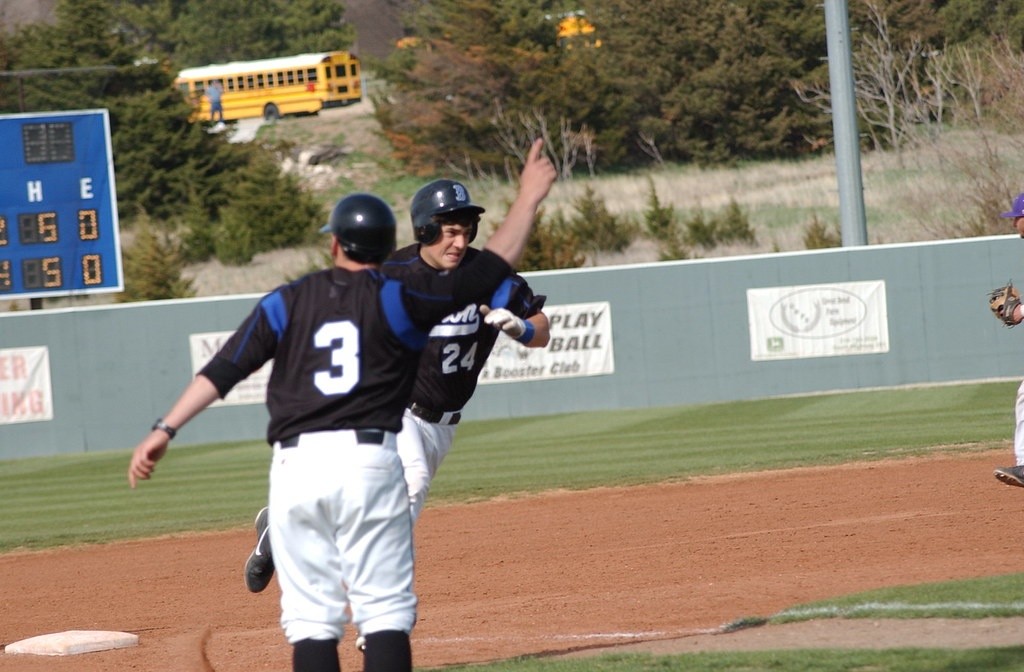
[205,80,224,120]
[990,193,1024,486]
[244,177,551,650]
[128,137,558,672]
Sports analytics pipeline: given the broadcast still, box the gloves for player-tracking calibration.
[480,304,529,341]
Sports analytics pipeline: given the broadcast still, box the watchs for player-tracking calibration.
[152,419,176,439]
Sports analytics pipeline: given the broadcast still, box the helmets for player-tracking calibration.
[318,193,397,260]
[410,179,485,247]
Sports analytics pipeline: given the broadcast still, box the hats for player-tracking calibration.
[1001,193,1024,217]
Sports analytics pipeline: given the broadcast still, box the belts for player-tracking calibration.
[280,429,384,448]
[406,399,460,425]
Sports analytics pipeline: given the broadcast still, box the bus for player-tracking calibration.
[396,10,604,73]
[172,50,362,123]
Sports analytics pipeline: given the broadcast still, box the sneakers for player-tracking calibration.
[245,505,275,592]
[993,465,1024,486]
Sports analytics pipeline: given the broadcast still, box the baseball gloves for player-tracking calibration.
[989,285,1022,327]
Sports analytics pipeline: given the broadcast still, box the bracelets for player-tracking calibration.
[516,320,534,344]
